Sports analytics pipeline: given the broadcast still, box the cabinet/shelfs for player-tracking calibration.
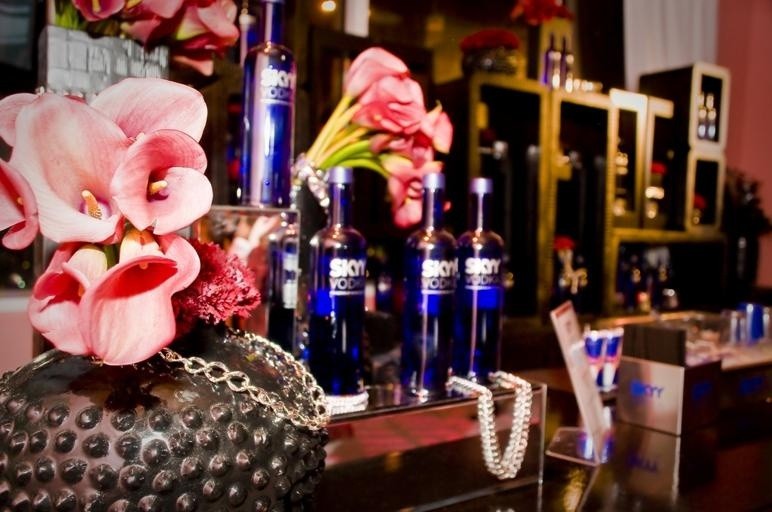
[608,63,732,317]
[549,89,616,318]
[435,72,549,335]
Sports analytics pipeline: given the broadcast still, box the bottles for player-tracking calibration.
[404,171,452,398]
[717,302,772,349]
[544,29,576,93]
[697,90,716,139]
[309,165,368,394]
[454,174,509,388]
[239,0,293,210]
[263,211,297,359]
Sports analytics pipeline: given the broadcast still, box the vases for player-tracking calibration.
[0,328,332,512]
[461,47,517,75]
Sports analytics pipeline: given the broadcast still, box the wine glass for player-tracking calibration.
[581,325,624,392]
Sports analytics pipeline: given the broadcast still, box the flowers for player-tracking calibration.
[0,77,264,368]
[54,0,241,77]
[460,26,521,54]
[304,47,454,230]
